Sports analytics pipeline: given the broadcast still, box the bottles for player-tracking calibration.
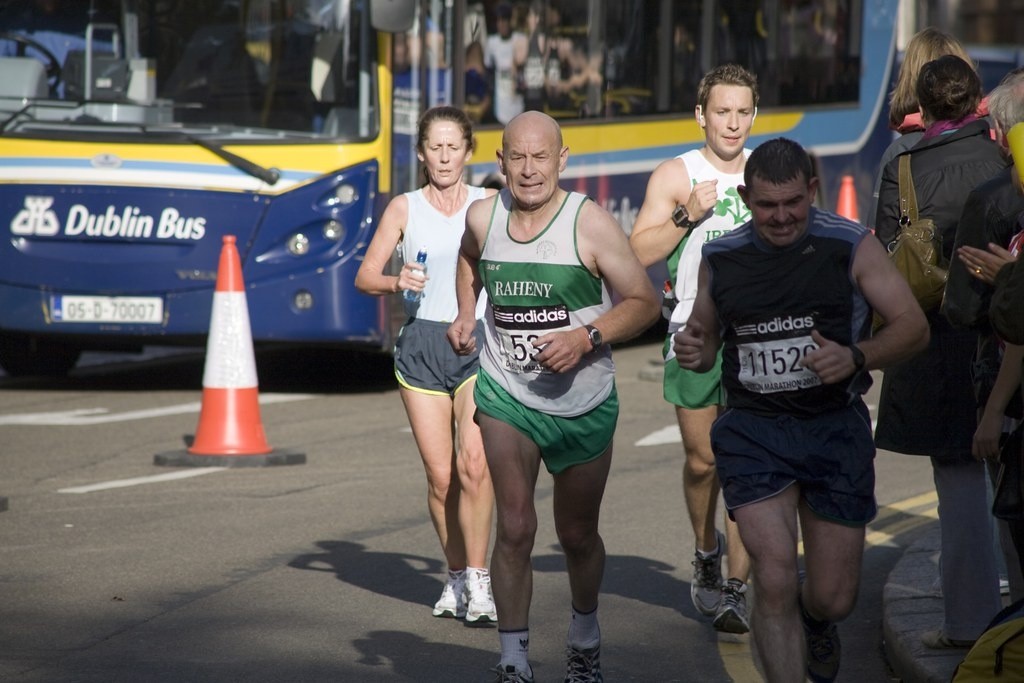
[402,249,427,303]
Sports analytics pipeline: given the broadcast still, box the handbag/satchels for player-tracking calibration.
[871,218,949,332]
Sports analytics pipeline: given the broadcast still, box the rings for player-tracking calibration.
[976,267,982,274]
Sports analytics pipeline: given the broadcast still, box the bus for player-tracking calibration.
[1,0,1024,380]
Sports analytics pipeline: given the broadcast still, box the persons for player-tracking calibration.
[459,0,840,124]
[673,137,931,683]
[629,64,758,635]
[354,105,500,628]
[445,111,661,683]
[863,30,1024,683]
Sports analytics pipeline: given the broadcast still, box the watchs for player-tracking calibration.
[847,343,866,371]
[672,205,700,228]
[584,325,602,352]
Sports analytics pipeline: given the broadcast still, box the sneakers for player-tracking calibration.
[690,528,726,616]
[564,620,604,683]
[464,570,497,622]
[921,628,976,650]
[490,662,535,683]
[714,578,751,636]
[798,568,842,683]
[432,569,467,618]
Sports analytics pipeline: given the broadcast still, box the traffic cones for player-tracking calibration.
[151,234,308,470]
[832,174,861,222]
